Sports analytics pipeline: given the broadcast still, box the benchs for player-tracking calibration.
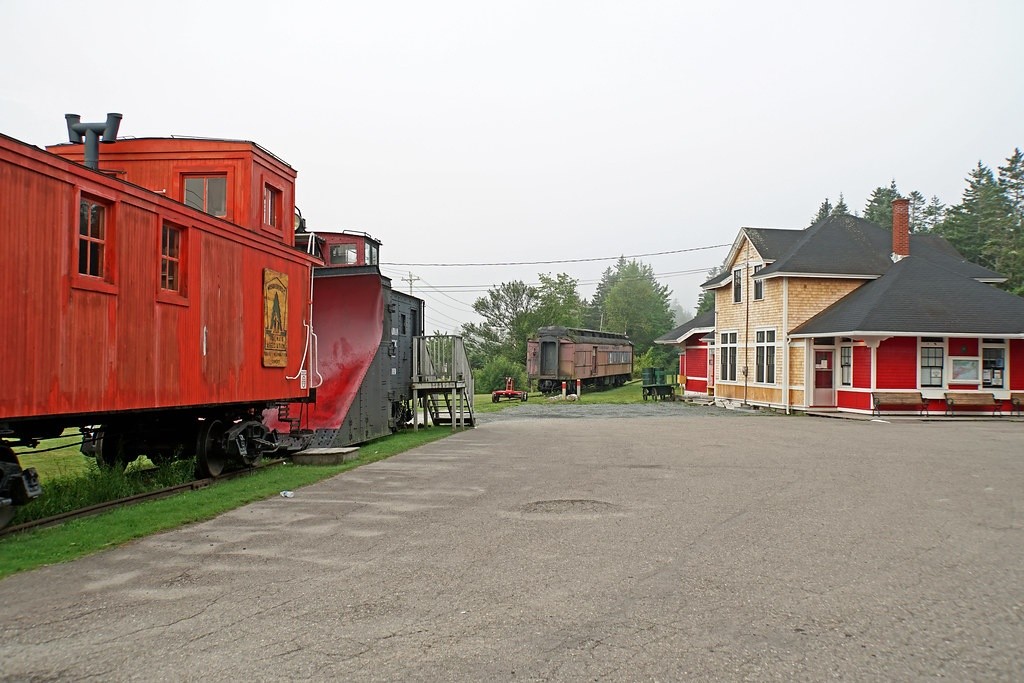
[944,393,1002,418]
[1011,393,1024,417]
[871,392,929,417]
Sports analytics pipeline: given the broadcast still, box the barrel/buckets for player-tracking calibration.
[654,368,664,384]
[642,368,653,384]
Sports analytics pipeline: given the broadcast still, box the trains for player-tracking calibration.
[0,112,426,535]
[526,326,634,395]
